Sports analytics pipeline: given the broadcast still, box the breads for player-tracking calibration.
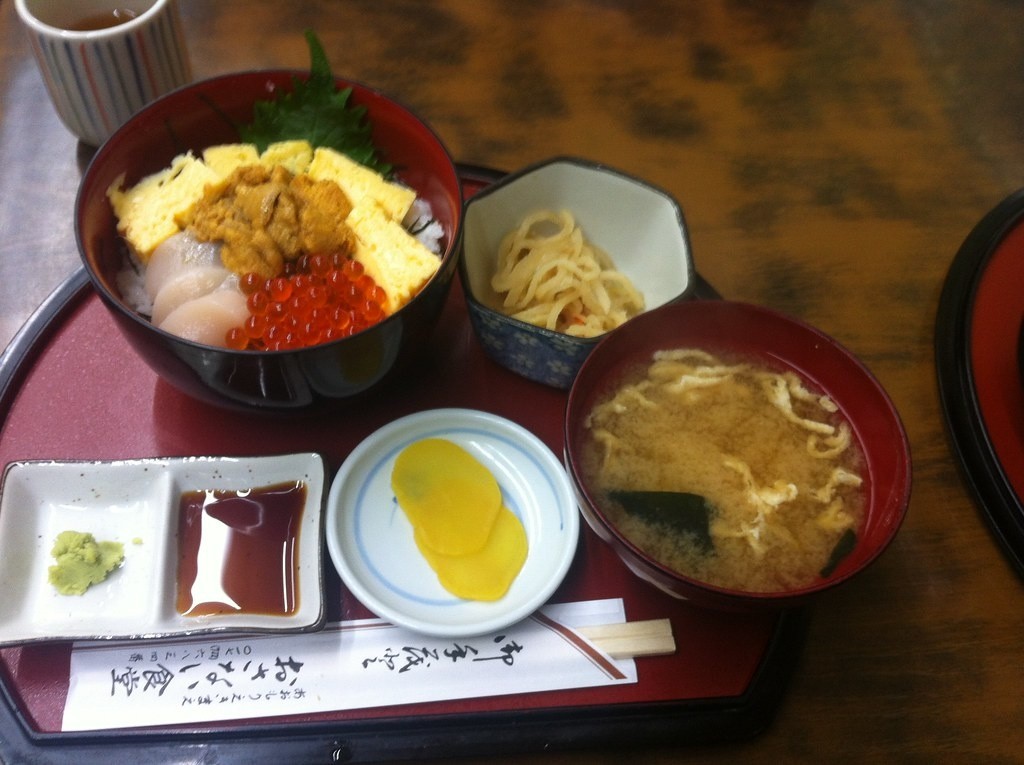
[106,140,442,312]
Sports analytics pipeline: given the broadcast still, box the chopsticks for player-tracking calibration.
[569,618,676,659]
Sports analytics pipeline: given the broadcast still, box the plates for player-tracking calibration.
[325,408,579,639]
[0,453,325,649]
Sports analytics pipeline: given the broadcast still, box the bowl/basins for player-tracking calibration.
[561,300,912,598]
[73,68,462,415]
[462,155,696,391]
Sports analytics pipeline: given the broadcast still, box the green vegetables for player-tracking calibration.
[195,24,395,181]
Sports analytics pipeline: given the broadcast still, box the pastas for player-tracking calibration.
[486,211,642,331]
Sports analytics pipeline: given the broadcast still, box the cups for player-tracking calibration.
[14,0,191,149]
